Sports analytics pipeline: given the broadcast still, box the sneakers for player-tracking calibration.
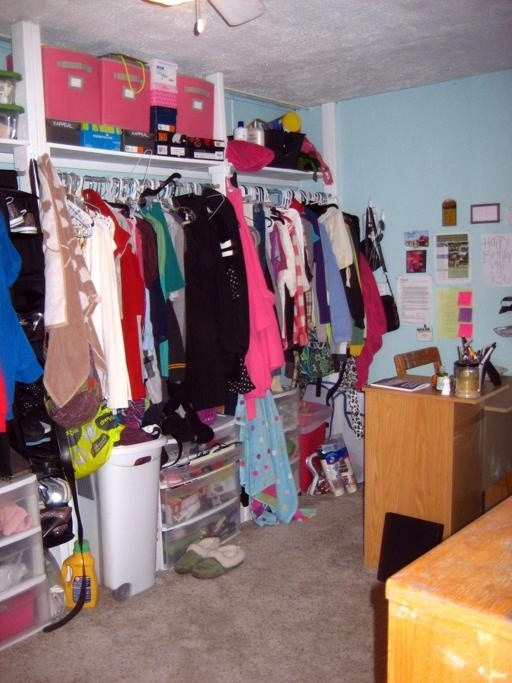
[191,545,244,579]
[175,537,221,573]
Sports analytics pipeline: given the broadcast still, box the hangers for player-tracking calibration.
[56,175,227,221]
[239,180,339,230]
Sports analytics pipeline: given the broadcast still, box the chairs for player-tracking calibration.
[394,345,443,377]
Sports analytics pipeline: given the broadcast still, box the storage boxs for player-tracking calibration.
[1,36,224,164]
[156,410,242,571]
[299,401,332,492]
[1,473,53,650]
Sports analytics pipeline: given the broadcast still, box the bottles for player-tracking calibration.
[453,360,480,399]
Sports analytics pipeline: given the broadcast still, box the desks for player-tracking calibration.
[384,494,512,683]
[362,373,512,572]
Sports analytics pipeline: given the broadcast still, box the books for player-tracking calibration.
[370,378,430,392]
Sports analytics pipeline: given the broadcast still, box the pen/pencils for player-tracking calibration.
[457,342,497,364]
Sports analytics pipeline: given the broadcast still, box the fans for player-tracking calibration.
[143,0,266,26]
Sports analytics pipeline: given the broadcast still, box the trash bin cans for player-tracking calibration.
[74,435,167,601]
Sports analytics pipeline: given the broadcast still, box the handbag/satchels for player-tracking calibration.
[360,238,381,271]
[381,296,400,331]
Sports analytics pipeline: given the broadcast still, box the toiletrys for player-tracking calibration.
[234,119,249,142]
[250,119,265,146]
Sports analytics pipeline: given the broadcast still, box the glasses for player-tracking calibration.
[376,220,384,242]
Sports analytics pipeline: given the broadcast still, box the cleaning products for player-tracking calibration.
[60,538,97,608]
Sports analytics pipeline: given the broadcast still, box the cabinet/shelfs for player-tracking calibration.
[0,22,338,432]
[242,383,302,523]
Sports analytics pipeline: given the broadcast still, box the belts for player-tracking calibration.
[43,425,86,632]
